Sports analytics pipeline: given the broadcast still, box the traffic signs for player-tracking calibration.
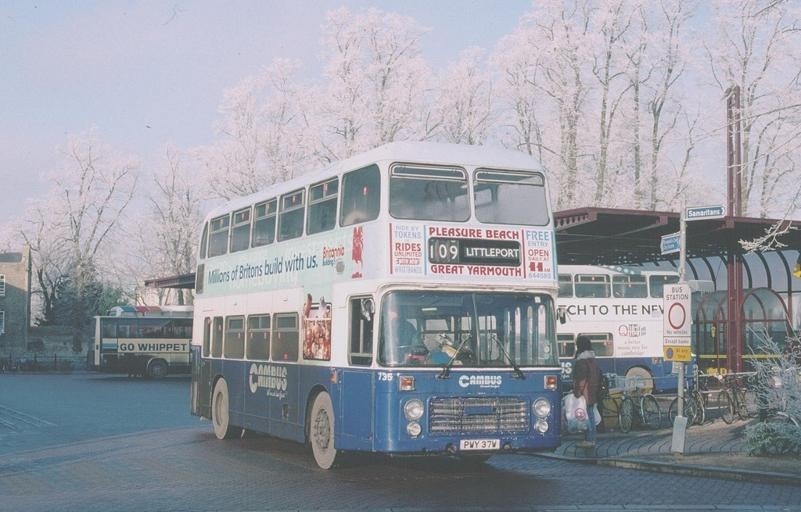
[686,205,727,221]
[660,231,681,256]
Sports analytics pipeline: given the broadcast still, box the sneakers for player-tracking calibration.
[575,440,595,449]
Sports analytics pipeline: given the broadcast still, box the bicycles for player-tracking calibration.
[603,374,752,433]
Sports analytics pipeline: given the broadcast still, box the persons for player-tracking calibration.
[572,335,599,448]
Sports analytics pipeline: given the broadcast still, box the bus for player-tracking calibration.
[87,304,195,379]
[194,140,565,470]
[407,265,696,402]
